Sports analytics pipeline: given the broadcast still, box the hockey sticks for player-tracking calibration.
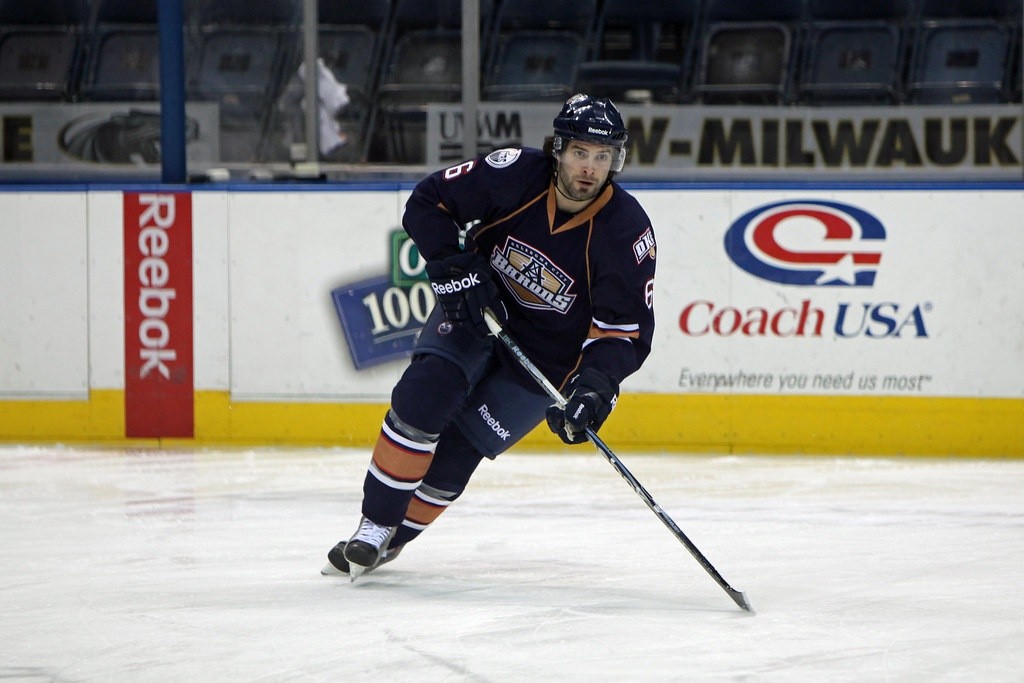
[482,310,752,616]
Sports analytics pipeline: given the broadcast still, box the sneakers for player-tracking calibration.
[344,514,396,582]
[321,539,405,575]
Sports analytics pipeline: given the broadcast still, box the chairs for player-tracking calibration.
[0,0,1011,170]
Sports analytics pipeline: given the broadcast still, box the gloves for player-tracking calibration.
[425,253,508,344]
[545,371,621,445]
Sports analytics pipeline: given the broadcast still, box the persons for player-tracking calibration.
[322,89,656,582]
[296,54,365,164]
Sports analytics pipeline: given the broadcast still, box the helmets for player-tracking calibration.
[553,93,628,172]
